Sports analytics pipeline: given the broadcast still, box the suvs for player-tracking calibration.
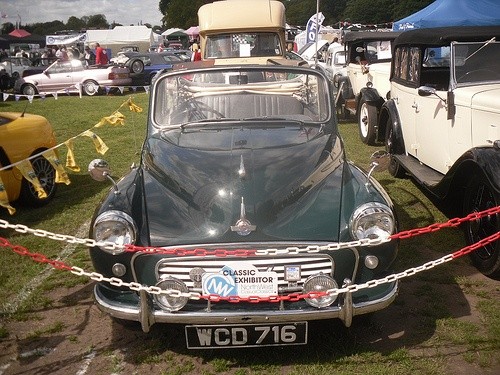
[337,31,402,144]
[377,27,499,277]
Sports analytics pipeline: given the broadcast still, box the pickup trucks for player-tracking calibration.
[317,46,347,89]
[13,58,132,100]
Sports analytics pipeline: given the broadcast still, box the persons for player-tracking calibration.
[7,39,245,66]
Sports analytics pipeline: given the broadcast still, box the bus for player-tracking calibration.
[198,0,287,60]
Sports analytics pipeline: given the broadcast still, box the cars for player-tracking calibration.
[89,64,401,349]
[0,45,195,93]
[0,113,59,205]
